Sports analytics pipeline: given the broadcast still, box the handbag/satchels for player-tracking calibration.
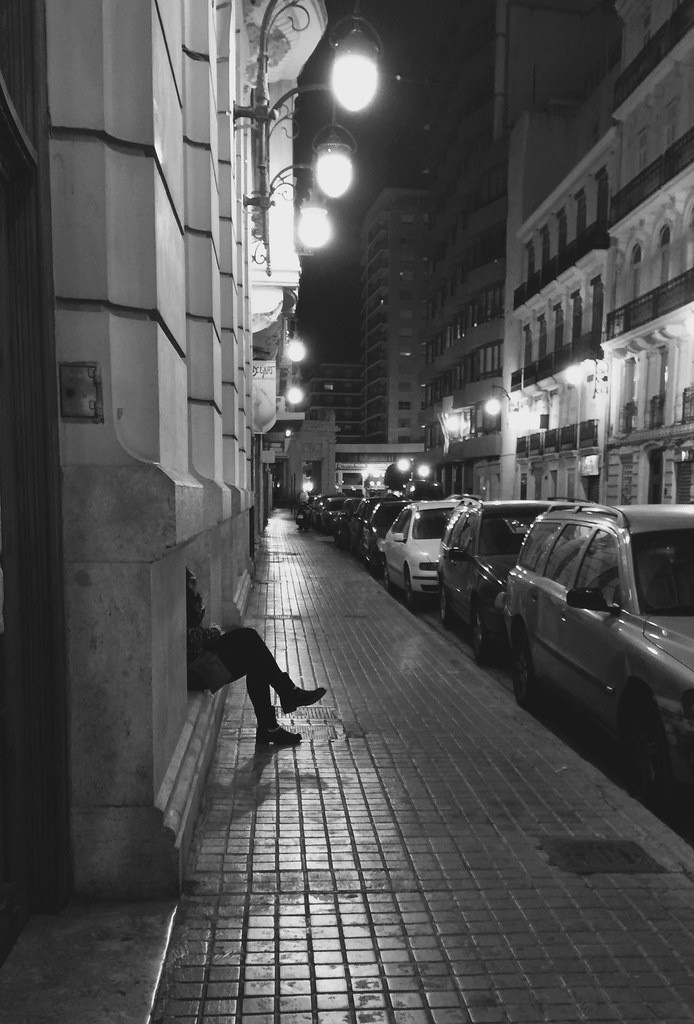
[187,651,234,695]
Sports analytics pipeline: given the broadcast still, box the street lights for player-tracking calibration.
[398,459,430,499]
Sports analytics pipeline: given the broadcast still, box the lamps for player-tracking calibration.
[218,0,384,277]
[485,385,512,416]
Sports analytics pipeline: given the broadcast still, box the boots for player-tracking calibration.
[270,673,327,714]
[254,706,302,745]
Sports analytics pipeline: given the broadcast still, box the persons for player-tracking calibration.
[186,567,327,745]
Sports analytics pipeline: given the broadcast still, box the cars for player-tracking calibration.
[319,497,347,535]
[312,494,349,530]
[358,501,419,569]
[439,496,597,667]
[364,476,385,488]
[330,498,364,550]
[381,499,462,609]
[344,497,399,558]
[373,492,397,499]
[446,493,483,504]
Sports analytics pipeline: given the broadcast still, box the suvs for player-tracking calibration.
[494,502,694,810]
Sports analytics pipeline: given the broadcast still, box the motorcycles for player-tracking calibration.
[295,501,312,531]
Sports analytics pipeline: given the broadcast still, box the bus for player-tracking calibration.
[336,470,366,498]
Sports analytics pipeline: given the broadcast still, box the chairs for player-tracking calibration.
[639,554,679,609]
[483,520,518,555]
